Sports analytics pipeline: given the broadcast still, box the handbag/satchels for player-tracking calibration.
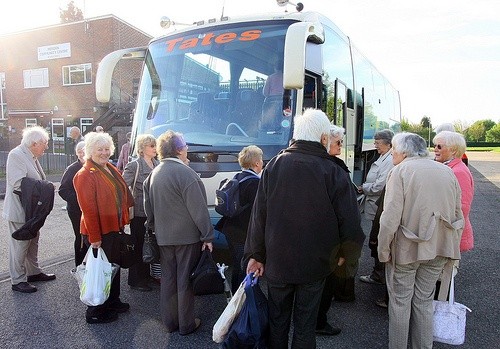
[212,273,251,343]
[143,227,160,263]
[70,244,120,306]
[128,160,139,220]
[220,272,269,349]
[433,265,472,345]
[114,228,139,269]
[191,246,224,296]
[149,263,162,280]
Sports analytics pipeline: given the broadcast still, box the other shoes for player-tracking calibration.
[314,323,341,335]
[360,275,382,284]
[131,284,152,291]
[195,318,200,328]
[375,298,387,307]
[146,276,160,286]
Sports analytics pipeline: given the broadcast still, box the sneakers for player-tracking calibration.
[110,299,130,312]
[86,309,118,323]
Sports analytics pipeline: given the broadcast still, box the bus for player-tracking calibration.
[95,0,403,217]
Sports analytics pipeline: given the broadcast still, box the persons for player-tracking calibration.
[2,126,57,294]
[59,127,190,323]
[214,146,263,296]
[314,123,474,349]
[241,110,362,349]
[143,131,216,335]
[263,60,283,97]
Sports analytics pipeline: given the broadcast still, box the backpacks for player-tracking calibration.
[215,172,259,218]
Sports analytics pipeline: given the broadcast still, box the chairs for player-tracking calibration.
[188,87,283,135]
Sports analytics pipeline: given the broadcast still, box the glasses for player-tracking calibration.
[144,145,154,148]
[434,144,447,150]
[330,140,343,145]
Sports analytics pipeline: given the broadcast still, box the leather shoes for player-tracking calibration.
[27,273,56,282]
[12,282,37,292]
